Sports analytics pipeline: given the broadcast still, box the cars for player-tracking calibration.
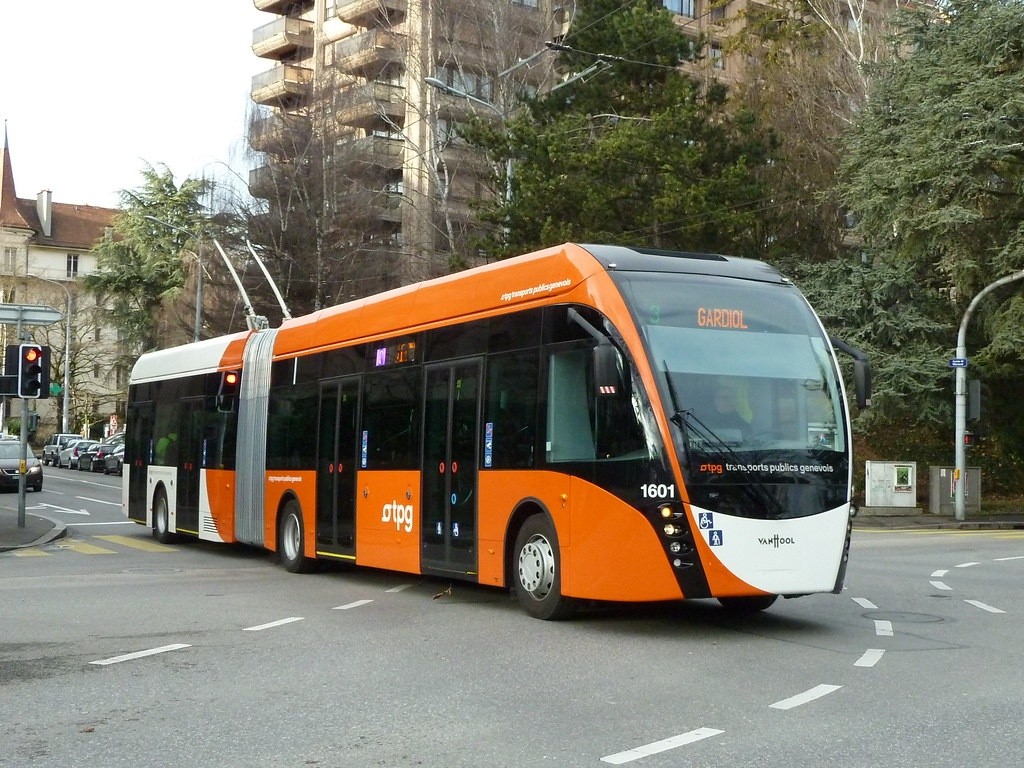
[54,433,125,475]
[0,433,43,492]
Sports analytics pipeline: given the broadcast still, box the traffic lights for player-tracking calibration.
[18,344,50,400]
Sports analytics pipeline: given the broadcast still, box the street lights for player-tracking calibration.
[423,76,515,244]
[26,271,71,433]
[143,215,202,342]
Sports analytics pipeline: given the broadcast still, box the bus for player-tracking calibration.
[122,229,873,622]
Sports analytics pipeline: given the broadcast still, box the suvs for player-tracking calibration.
[42,434,83,467]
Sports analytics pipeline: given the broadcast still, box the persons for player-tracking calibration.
[154,426,177,465]
[705,387,750,437]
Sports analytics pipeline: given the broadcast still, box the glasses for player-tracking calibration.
[715,395,738,402]
